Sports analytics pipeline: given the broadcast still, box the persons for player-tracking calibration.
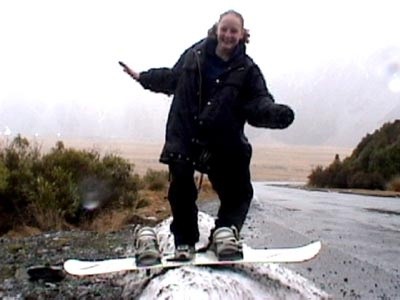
[117,10,294,261]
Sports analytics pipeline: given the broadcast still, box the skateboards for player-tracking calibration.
[64,241,322,276]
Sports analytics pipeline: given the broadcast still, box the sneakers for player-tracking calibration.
[212,225,239,255]
[175,245,193,259]
